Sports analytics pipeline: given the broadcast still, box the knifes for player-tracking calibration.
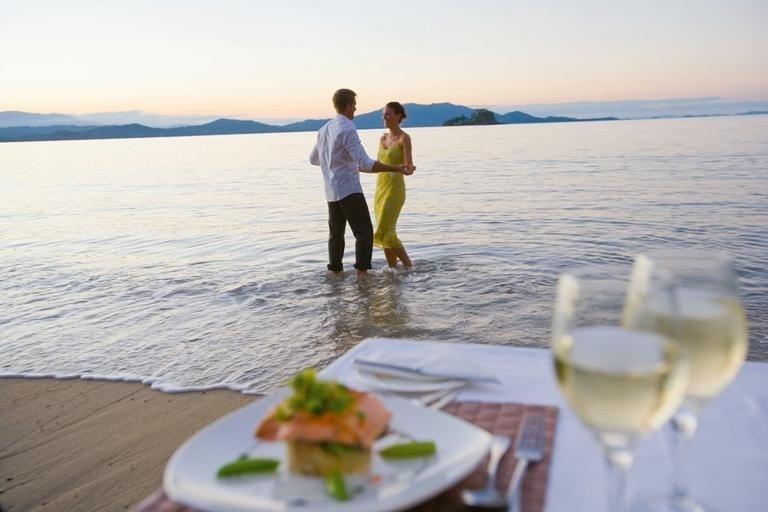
[353,359,505,386]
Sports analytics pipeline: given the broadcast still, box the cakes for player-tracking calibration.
[255,370,389,479]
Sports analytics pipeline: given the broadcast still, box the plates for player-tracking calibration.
[352,348,481,393]
[159,381,493,512]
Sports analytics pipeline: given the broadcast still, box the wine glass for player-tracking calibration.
[617,246,750,512]
[548,259,695,512]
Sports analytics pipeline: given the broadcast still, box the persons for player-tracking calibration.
[309,88,416,277]
[373,102,416,269]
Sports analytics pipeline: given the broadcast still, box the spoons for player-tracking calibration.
[459,433,511,511]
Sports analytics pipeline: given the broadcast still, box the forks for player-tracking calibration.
[502,408,546,512]
